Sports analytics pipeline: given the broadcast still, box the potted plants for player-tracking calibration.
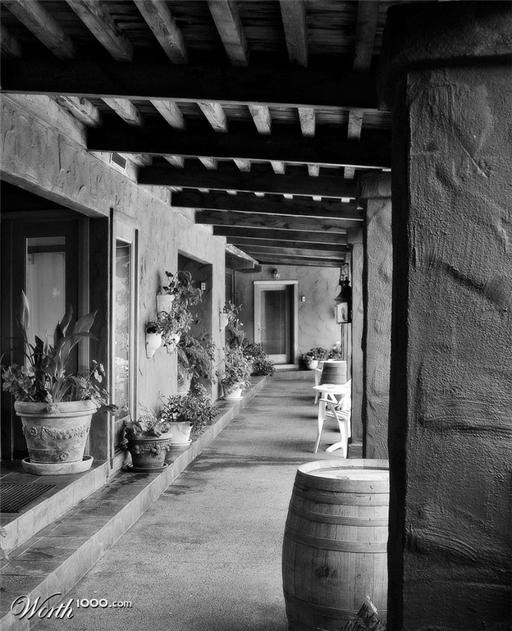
[120,271,273,470]
[0,291,116,476]
[303,348,326,369]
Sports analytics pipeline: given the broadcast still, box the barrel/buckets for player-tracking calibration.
[319,361,346,410]
[282,458,390,631]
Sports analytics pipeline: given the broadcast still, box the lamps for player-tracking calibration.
[335,278,351,324]
[273,268,280,279]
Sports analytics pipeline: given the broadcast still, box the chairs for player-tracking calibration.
[314,391,351,458]
[314,379,352,403]
[315,361,324,404]
